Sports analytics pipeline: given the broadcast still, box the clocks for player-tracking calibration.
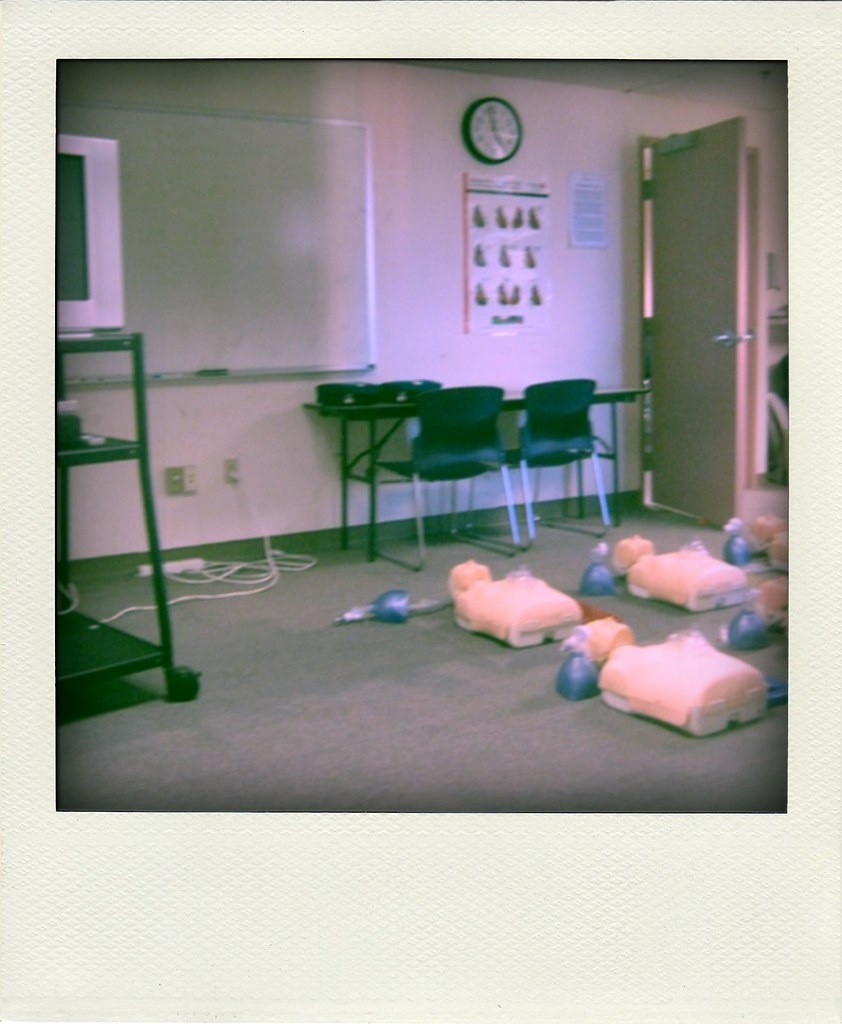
[462,96,522,165]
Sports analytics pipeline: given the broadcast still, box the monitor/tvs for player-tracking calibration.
[56,135,126,339]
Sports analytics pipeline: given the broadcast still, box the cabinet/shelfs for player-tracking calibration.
[56,330,201,724]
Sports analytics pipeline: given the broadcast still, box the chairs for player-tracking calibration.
[466,378,609,552]
[373,386,522,571]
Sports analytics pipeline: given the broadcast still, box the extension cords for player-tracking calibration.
[137,558,202,576]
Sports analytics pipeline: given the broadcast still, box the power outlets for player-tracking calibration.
[224,458,241,484]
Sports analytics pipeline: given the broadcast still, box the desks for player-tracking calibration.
[303,385,652,561]
